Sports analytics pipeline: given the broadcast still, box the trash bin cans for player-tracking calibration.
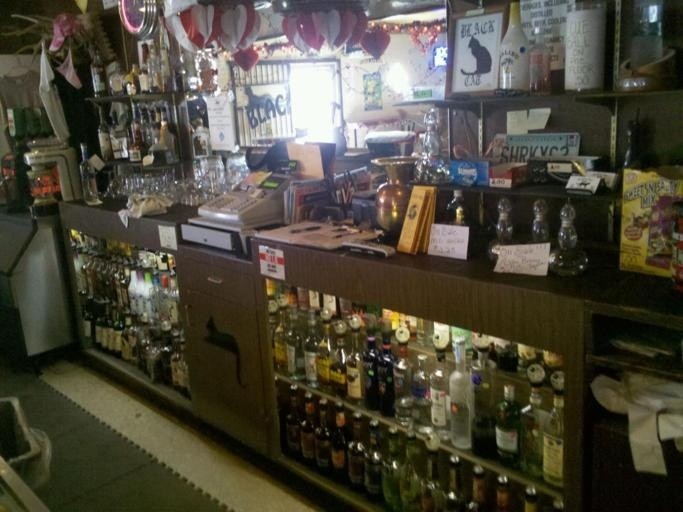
[0,397,41,500]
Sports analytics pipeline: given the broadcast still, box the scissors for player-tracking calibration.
[319,174,337,203]
[454,142,503,162]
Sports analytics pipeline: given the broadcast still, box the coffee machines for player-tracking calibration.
[22,137,81,218]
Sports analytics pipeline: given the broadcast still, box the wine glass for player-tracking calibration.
[118,170,222,208]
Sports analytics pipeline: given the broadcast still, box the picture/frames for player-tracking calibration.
[443,3,509,100]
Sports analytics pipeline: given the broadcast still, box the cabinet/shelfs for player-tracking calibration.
[179,244,268,449]
[400,1,682,277]
[85,1,193,208]
[59,199,181,402]
[253,236,587,509]
[587,296,683,510]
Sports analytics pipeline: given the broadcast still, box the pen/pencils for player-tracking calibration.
[331,182,355,203]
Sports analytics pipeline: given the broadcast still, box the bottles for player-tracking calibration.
[564,0,603,91]
[488,197,524,264]
[95,102,180,165]
[445,189,472,228]
[526,199,588,278]
[268,281,565,512]
[70,230,191,399]
[497,0,552,94]
[416,111,453,186]
[193,118,248,192]
[79,143,98,201]
[90,43,218,95]
